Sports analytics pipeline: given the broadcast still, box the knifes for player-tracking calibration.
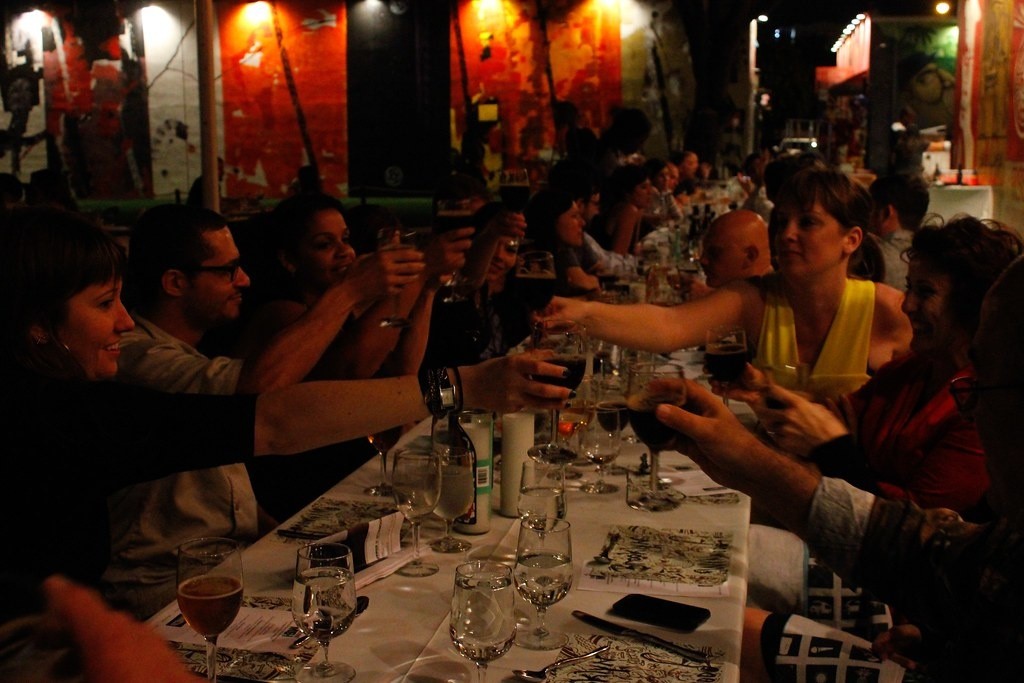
[572,610,708,663]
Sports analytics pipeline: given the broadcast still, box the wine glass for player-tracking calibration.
[622,348,687,511]
[703,324,747,406]
[513,517,574,650]
[518,459,567,549]
[435,198,475,302]
[718,174,730,198]
[291,542,358,683]
[558,372,643,495]
[449,560,518,683]
[363,425,403,497]
[530,318,587,463]
[516,249,558,337]
[175,536,245,683]
[377,226,417,328]
[499,167,532,246]
[392,447,472,577]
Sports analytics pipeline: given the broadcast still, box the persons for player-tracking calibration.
[0,206,579,628]
[1,100,1024,683]
[704,210,1024,526]
[530,170,912,408]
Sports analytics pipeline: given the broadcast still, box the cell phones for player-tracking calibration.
[612,593,711,632]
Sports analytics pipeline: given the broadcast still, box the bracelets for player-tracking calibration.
[417,367,448,420]
[453,366,464,412]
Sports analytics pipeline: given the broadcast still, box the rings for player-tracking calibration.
[765,420,786,442]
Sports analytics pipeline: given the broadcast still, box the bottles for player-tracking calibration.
[635,202,713,258]
[591,288,621,374]
[435,410,494,535]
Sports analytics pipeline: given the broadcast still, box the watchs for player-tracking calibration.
[437,368,456,410]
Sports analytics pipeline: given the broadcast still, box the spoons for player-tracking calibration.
[511,645,612,683]
[290,595,370,649]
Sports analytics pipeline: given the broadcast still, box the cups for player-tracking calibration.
[759,361,807,408]
[594,266,622,291]
[676,260,700,275]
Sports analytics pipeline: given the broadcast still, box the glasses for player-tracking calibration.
[587,199,603,209]
[194,263,239,282]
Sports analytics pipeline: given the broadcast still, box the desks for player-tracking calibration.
[841,163,995,231]
[131,228,759,683]
